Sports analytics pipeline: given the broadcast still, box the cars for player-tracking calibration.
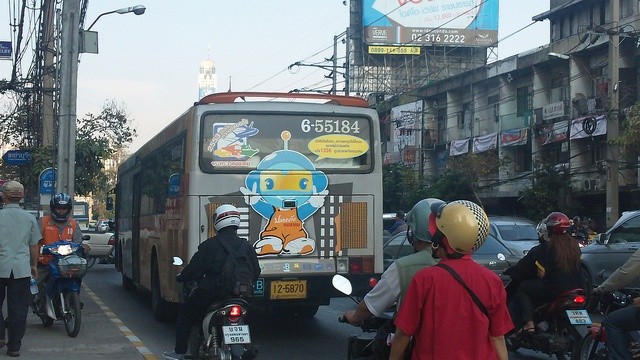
[383,230,521,287]
[90,217,115,234]
[580,210,640,313]
[382,212,407,231]
[488,215,543,260]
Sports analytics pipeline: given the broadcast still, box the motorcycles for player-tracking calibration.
[29,235,90,337]
[330,271,394,360]
[574,268,640,359]
[171,255,260,359]
[497,249,592,360]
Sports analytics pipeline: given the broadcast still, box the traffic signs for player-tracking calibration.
[2,149,35,166]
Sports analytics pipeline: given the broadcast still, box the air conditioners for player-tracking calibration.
[583,179,600,191]
[598,162,607,173]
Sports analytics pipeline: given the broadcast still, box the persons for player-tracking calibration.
[342,197,477,359]
[37,192,92,313]
[515,211,583,338]
[597,246,640,360]
[0,181,43,358]
[390,209,406,235]
[163,203,261,360]
[503,219,550,313]
[568,215,589,246]
[389,199,515,360]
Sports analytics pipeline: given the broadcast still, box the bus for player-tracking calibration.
[105,90,385,323]
[73,201,89,231]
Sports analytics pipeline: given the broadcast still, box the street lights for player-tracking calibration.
[546,50,613,108]
[86,5,147,30]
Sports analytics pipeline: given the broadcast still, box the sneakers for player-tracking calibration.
[0,339,6,349]
[7,349,21,357]
[163,350,184,360]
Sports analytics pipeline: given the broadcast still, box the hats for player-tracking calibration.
[3,180,25,200]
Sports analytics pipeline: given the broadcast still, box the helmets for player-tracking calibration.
[213,205,240,231]
[544,212,570,234]
[431,200,490,255]
[573,216,581,224]
[536,219,551,241]
[50,193,73,222]
[404,198,444,243]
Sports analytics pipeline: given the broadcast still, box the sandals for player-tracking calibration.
[516,326,536,336]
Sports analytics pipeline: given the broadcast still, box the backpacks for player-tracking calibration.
[216,235,254,303]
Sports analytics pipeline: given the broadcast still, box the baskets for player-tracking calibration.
[56,257,88,278]
